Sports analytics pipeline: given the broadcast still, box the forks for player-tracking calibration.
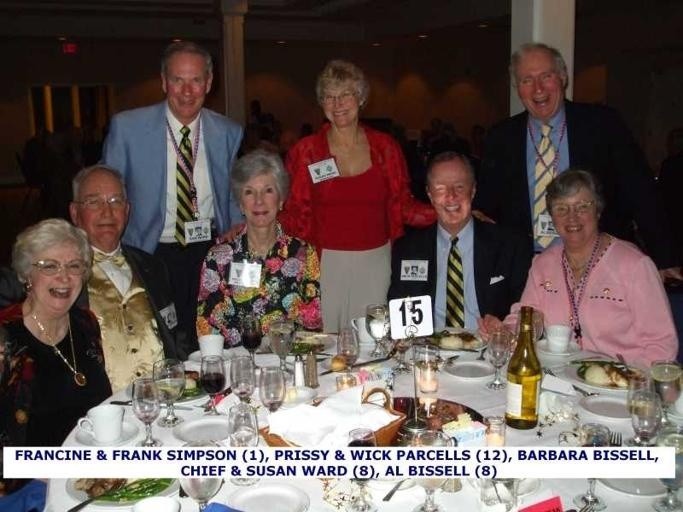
[578,504,594,512]
[610,431,622,446]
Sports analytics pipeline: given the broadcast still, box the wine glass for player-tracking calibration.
[487,328,508,392]
[650,426,683,512]
[573,419,610,512]
[364,302,390,359]
[115,320,297,511]
[382,427,462,511]
[348,428,376,512]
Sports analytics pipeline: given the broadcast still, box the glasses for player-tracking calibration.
[319,92,355,106]
[31,258,87,276]
[553,199,595,218]
[75,196,125,210]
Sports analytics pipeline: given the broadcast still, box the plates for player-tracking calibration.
[442,361,499,382]
[594,479,667,498]
[563,356,649,394]
[439,327,490,352]
[265,331,335,357]
[74,422,139,448]
[578,392,635,420]
[66,453,184,508]
[535,338,581,356]
[227,479,310,512]
[466,478,541,497]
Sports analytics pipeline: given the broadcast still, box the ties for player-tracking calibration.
[173,125,194,249]
[445,236,464,328]
[534,123,558,249]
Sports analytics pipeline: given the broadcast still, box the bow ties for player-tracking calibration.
[92,251,125,268]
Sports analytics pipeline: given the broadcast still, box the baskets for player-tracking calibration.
[260,387,407,449]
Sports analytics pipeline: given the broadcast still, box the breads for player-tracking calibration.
[332,355,347,370]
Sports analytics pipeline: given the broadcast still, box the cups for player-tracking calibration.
[77,404,122,440]
[350,315,379,343]
[544,323,571,353]
[414,344,439,392]
[620,361,682,449]
[334,329,359,379]
[478,475,516,511]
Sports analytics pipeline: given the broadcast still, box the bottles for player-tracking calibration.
[503,305,544,431]
[482,414,505,448]
[294,350,319,390]
